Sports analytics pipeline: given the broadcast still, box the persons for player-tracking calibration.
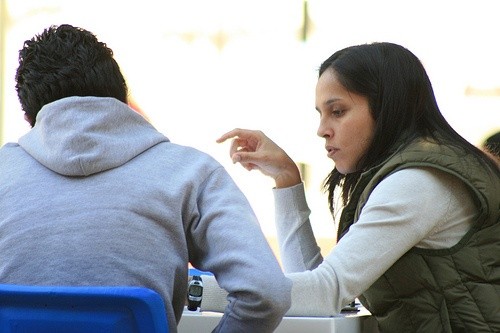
[182,42,500,333]
[0,23,293,333]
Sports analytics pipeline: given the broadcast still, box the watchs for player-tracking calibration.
[187,275,203,311]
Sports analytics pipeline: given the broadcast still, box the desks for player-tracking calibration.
[177,297,375,333]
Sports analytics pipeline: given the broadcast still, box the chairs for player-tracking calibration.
[0,283,169,333]
